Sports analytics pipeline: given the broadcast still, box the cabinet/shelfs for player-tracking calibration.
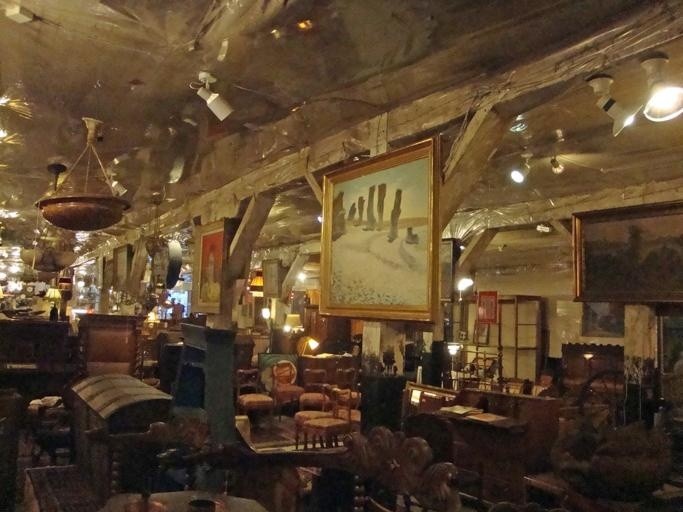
[437,291,549,389]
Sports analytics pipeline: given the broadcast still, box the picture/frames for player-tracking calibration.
[262,258,283,298]
[571,198,683,308]
[438,235,455,303]
[317,135,445,325]
[190,215,232,316]
[112,244,132,290]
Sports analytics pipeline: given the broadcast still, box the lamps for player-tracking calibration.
[585,73,644,139]
[637,51,682,121]
[510,152,534,183]
[44,287,62,319]
[548,130,568,174]
[188,70,235,122]
[249,269,264,293]
[107,161,128,196]
[282,313,305,334]
